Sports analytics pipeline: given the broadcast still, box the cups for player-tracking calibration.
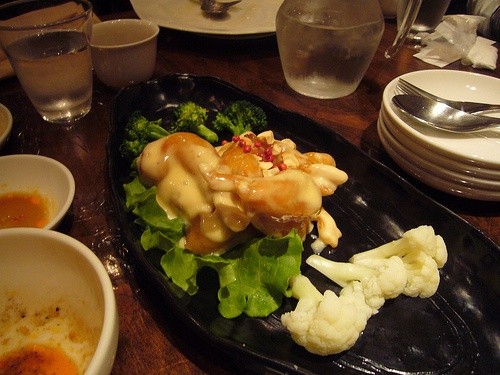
[0,0,94,126]
[405,0,451,49]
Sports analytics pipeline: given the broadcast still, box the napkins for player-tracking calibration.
[412,15,498,71]
[0,2,103,77]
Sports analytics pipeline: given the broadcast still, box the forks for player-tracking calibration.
[395,77,500,115]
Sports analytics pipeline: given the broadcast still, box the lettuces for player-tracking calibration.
[123,174,304,317]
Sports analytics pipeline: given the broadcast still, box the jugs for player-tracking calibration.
[275,1,423,101]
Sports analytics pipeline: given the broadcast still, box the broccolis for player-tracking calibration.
[279,223,448,355]
[118,99,267,158]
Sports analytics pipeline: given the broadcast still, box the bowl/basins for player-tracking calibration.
[0,153,77,230]
[0,227,120,375]
[86,19,161,91]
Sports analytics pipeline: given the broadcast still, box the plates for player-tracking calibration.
[377,68,500,203]
[0,101,13,145]
[127,0,288,41]
[105,72,500,375]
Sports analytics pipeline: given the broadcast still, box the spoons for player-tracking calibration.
[394,94,500,134]
